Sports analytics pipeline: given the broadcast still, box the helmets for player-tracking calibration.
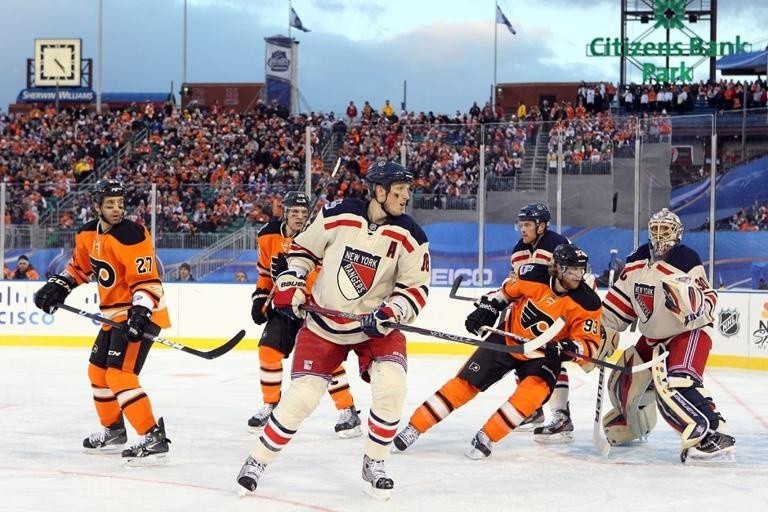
[515,203,551,232]
[647,208,684,256]
[91,179,127,208]
[364,160,414,196]
[553,243,592,280]
[280,192,313,208]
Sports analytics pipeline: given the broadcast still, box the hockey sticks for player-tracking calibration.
[56,301,245,360]
[261,156,341,316]
[593,250,617,458]
[298,304,566,354]
[450,276,478,301]
[479,325,670,374]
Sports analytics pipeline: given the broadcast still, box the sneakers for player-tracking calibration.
[362,453,394,489]
[394,422,421,451]
[471,429,492,457]
[237,453,267,491]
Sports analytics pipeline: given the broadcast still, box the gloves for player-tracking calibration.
[465,296,502,338]
[252,288,274,325]
[275,270,307,321]
[35,274,74,315]
[545,338,576,360]
[119,305,152,342]
[361,300,403,337]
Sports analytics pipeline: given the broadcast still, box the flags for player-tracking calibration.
[289,5,313,33]
[497,6,518,36]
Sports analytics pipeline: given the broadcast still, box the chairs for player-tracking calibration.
[168,208,255,245]
[46,212,88,248]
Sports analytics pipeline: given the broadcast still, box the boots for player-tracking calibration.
[520,406,545,426]
[83,411,127,448]
[687,397,737,458]
[534,401,573,435]
[335,397,361,434]
[122,417,171,459]
[247,391,282,429]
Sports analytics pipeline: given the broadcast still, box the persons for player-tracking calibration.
[394,240,605,459]
[34,177,173,460]
[507,198,596,437]
[578,203,739,457]
[251,187,363,432]
[234,157,433,494]
[0,97,531,294]
[513,75,768,234]
